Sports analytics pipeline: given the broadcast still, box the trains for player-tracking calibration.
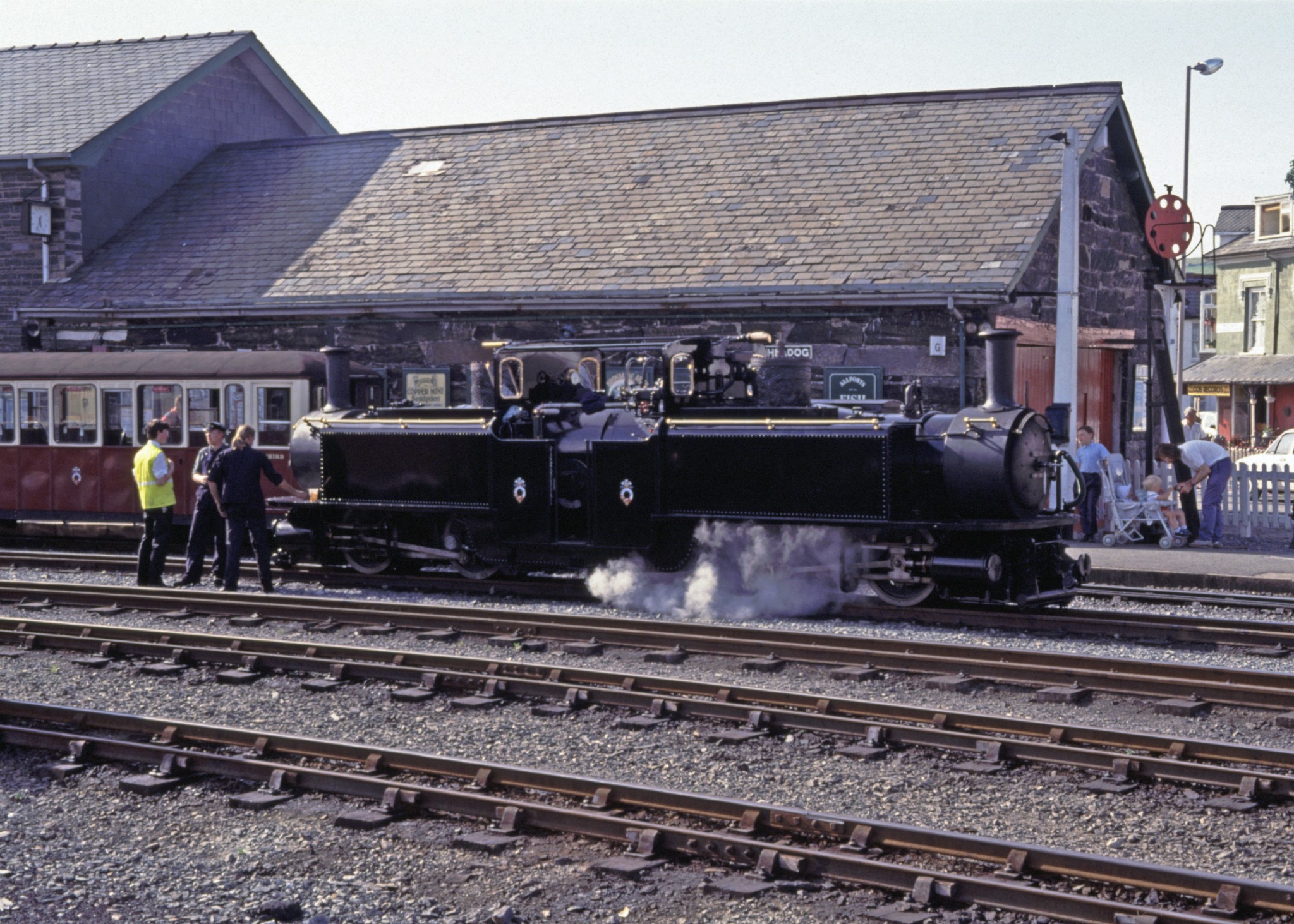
[260,329,1094,616]
[1,342,388,546]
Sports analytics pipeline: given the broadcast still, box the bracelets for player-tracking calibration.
[1190,481,1193,486]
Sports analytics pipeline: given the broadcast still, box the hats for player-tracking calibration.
[203,421,226,433]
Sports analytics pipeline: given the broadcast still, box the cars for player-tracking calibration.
[1233,428,1294,501]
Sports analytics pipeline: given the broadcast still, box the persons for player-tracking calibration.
[156,393,192,429]
[172,422,227,586]
[1074,407,1233,549]
[206,424,306,592]
[134,420,174,587]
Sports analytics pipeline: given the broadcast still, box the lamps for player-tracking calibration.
[1174,289,1182,303]
[1049,131,1069,145]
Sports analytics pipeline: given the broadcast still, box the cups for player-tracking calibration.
[308,488,319,502]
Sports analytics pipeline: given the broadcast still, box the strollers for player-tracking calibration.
[1095,452,1190,550]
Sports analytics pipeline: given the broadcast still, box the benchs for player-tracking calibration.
[0,420,207,447]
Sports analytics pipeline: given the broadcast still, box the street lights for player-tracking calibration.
[1176,57,1225,420]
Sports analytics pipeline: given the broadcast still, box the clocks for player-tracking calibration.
[20,199,53,236]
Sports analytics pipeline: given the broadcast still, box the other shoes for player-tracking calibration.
[1088,535,1096,543]
[1214,541,1223,549]
[1175,529,1189,537]
[212,575,223,585]
[1182,529,1193,537]
[1190,539,1214,548]
[172,578,201,587]
[1080,535,1089,542]
[147,578,169,587]
[136,577,148,585]
